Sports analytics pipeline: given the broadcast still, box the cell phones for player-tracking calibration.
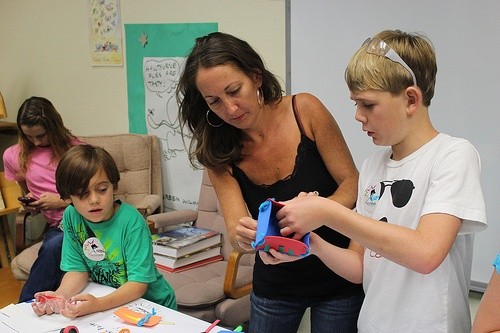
[18,196,36,206]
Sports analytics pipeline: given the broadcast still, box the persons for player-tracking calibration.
[259,29,489,333]
[3,96,85,304]
[470,251,500,333]
[31,145,177,319]
[176,32,365,333]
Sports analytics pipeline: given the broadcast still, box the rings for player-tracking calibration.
[313,191,319,196]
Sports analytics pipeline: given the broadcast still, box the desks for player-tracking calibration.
[0,282,230,333]
[0,170,21,266]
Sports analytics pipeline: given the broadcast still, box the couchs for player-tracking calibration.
[16,134,164,255]
[145,146,255,326]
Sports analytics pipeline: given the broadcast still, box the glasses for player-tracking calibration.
[362,37,417,87]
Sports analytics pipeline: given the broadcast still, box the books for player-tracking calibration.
[152,224,224,274]
